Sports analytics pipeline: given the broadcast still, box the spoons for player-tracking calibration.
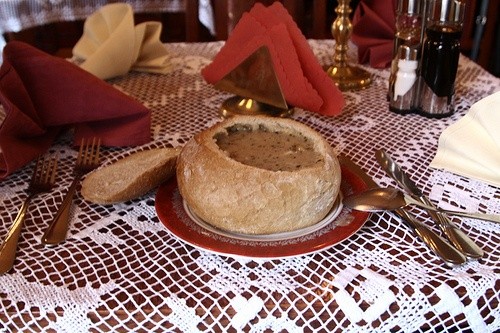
[342,188,500,223]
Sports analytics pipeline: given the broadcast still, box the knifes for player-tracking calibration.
[338,155,466,267]
[375,150,483,260]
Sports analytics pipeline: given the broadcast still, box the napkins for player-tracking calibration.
[0,3,174,179]
[201,0,397,116]
[429,90,500,188]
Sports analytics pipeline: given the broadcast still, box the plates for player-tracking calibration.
[155,155,370,259]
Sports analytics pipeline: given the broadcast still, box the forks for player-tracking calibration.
[0,151,59,276]
[41,137,101,246]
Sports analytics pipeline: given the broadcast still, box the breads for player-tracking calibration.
[174,115,341,234]
[79,147,181,204]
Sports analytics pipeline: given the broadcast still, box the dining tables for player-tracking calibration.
[0,39,500,333]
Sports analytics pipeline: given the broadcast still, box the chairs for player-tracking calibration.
[0,0,198,54]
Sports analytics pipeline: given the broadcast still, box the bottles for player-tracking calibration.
[415,0,466,118]
[389,0,428,114]
